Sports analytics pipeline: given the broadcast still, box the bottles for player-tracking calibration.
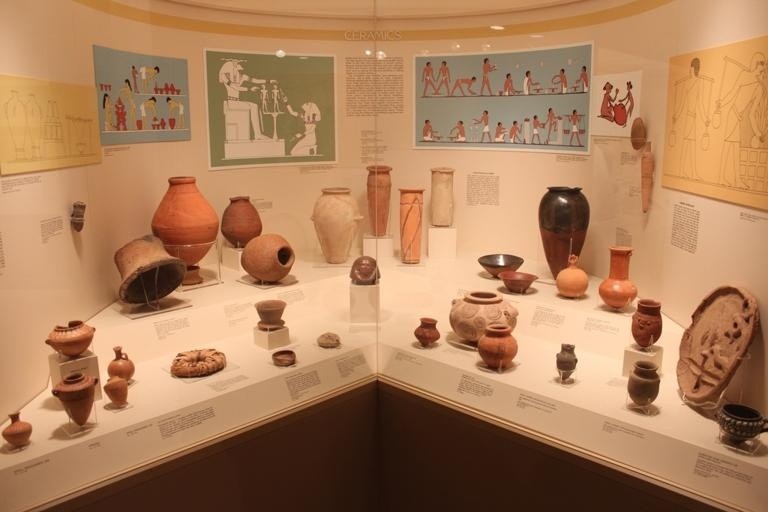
[219,197,262,249]
[365,163,393,241]
[254,297,286,331]
[429,166,457,228]
[497,270,540,294]
[412,317,442,350]
[478,323,518,374]
[555,341,578,382]
[272,349,294,369]
[539,186,590,283]
[397,187,424,265]
[449,291,519,345]
[151,177,220,268]
[477,251,525,282]
[715,403,768,445]
[555,254,589,300]
[49,372,101,430]
[240,232,296,282]
[626,359,660,406]
[629,300,663,352]
[102,375,131,412]
[310,186,365,266]
[113,233,188,306]
[598,245,638,313]
[2,412,35,445]
[347,254,381,287]
[45,320,96,359]
[105,346,135,383]
